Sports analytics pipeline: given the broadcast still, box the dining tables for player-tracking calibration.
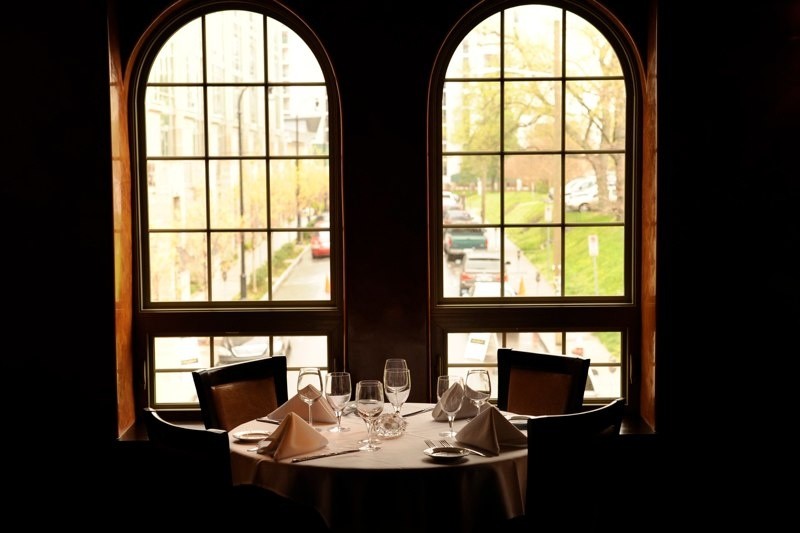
[227,398,537,533]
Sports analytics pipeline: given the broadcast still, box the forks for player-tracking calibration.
[424,440,437,448]
[439,440,486,456]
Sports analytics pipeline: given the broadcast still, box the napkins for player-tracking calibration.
[433,383,479,423]
[266,383,340,425]
[455,401,528,457]
[257,411,329,461]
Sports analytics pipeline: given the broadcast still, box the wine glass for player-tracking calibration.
[384,359,411,422]
[325,372,352,432]
[437,375,465,438]
[355,380,384,452]
[465,368,491,422]
[297,367,324,432]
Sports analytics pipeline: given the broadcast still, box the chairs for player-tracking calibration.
[143,406,330,533]
[191,356,289,433]
[525,397,626,533]
[497,348,591,417]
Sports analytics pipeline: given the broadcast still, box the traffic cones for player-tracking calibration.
[518,278,526,296]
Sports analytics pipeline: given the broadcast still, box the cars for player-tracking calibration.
[311,220,330,258]
[549,177,617,212]
[443,192,513,297]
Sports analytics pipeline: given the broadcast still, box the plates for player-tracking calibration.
[232,431,270,442]
[423,446,470,461]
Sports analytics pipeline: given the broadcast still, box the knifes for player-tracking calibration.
[292,449,360,463]
[401,407,434,417]
